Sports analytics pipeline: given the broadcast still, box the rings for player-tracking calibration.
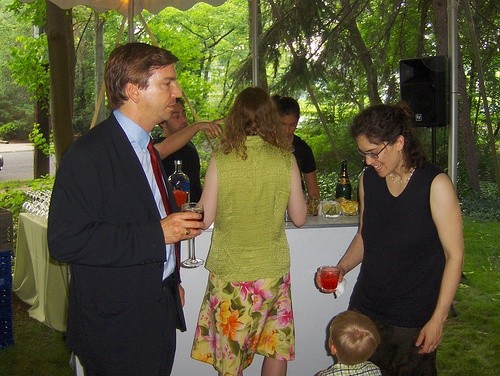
[321,287,323,291]
[186,228,191,235]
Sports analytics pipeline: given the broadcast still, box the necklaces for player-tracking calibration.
[390,168,416,184]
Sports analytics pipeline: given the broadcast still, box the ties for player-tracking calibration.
[147,136,182,285]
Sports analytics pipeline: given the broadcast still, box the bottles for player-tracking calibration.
[335,159,351,201]
[167,160,191,213]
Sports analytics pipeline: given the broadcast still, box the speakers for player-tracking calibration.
[398,55,450,127]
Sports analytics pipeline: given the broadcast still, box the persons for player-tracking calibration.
[47,42,206,376]
[272,94,320,199]
[190,86,307,376]
[314,101,464,376]
[151,97,204,222]
[315,310,382,376]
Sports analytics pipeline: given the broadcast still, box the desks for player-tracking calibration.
[15,214,70,329]
[76,213,361,376]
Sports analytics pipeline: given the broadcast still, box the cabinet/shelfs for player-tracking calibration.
[0,205,13,345]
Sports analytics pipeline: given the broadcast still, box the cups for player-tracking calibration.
[304,190,358,218]
[319,266,340,293]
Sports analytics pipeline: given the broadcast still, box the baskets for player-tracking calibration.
[0,207,15,346]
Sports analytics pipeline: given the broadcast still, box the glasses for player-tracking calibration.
[360,140,391,159]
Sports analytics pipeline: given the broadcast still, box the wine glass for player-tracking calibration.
[22,188,52,219]
[180,203,204,269]
